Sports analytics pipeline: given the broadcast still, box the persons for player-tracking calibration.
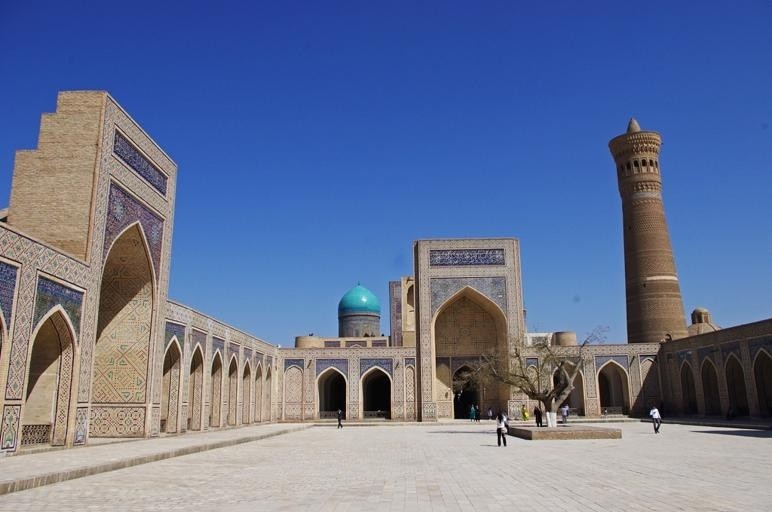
[474,404,480,422]
[560,405,568,423]
[496,411,509,446]
[521,404,529,421]
[336,408,343,428]
[503,411,510,435]
[469,403,476,422]
[564,403,570,416]
[534,406,543,427]
[649,406,661,433]
[487,408,493,420]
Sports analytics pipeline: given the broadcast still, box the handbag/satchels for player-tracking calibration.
[501,427,508,433]
